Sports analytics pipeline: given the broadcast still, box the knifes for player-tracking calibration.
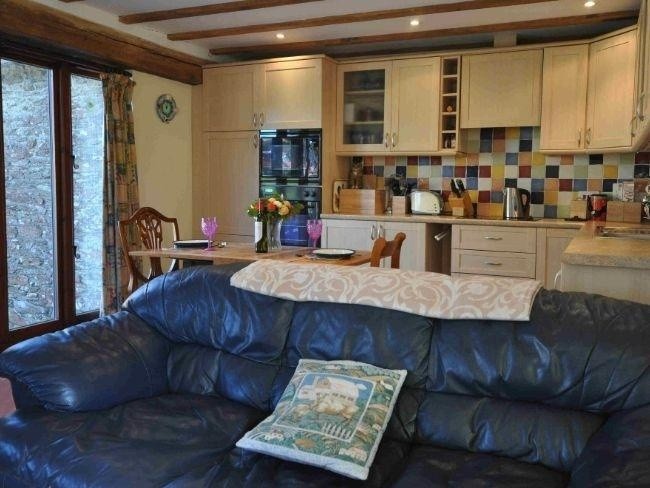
[449,178,465,198]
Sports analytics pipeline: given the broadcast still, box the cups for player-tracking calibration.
[349,134,378,144]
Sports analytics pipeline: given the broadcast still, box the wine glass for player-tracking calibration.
[201,216,217,251]
[305,219,323,253]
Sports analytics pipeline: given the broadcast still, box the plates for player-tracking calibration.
[311,248,356,259]
[173,239,214,247]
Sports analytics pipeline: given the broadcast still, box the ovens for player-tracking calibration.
[257,129,321,247]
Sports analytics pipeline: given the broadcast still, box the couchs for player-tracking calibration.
[0,264,650,488]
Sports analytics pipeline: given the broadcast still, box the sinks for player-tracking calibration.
[595,231,650,239]
[595,226,650,231]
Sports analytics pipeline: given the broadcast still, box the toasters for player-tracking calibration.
[408,188,444,215]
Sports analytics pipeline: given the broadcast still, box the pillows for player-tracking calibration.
[236,358,409,480]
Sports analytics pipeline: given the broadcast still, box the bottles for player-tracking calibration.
[363,106,375,121]
[253,198,268,254]
[446,104,454,112]
[500,185,531,220]
[443,133,455,148]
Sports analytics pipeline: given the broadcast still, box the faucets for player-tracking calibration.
[642,196,650,206]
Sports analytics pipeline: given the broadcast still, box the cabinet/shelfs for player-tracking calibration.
[206,58,337,131]
[207,131,258,245]
[321,218,650,304]
[335,54,462,156]
[540,0,649,154]
[460,46,542,129]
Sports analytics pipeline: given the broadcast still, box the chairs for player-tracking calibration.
[117,207,180,295]
